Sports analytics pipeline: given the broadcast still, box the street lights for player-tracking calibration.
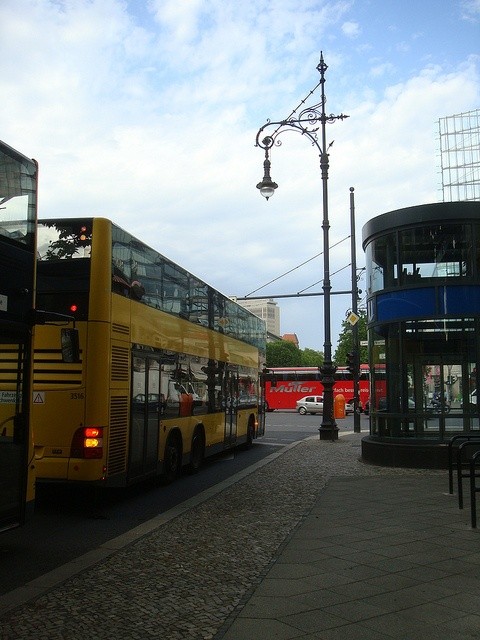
[254,51,348,440]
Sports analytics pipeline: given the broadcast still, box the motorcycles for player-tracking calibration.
[432,398,450,412]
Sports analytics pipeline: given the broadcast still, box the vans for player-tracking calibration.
[460,389,477,411]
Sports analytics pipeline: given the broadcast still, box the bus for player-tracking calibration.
[33,218,276,487]
[0,140,81,533]
[264,364,387,412]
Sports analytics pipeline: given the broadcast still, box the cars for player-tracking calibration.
[364,397,432,419]
[296,395,353,416]
